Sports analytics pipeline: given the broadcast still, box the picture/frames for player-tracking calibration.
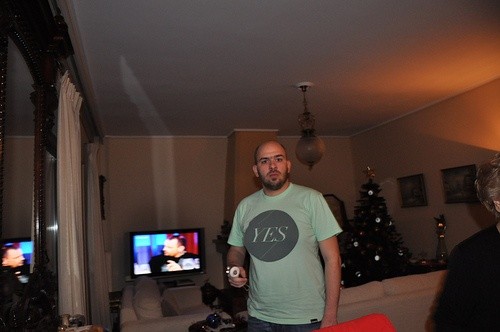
[396,173,429,208]
[440,164,475,205]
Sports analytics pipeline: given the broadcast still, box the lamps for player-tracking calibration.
[295,82,325,169]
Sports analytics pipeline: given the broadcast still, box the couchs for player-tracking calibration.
[119,277,212,332]
[337,270,447,332]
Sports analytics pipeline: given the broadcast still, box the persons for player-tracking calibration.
[426,153,500,332]
[225,140,343,332]
[147,235,198,267]
[2,242,27,269]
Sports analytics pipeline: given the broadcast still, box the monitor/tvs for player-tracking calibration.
[130,228,206,279]
[0,238,33,284]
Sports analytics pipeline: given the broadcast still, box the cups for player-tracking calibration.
[91,321,104,332]
